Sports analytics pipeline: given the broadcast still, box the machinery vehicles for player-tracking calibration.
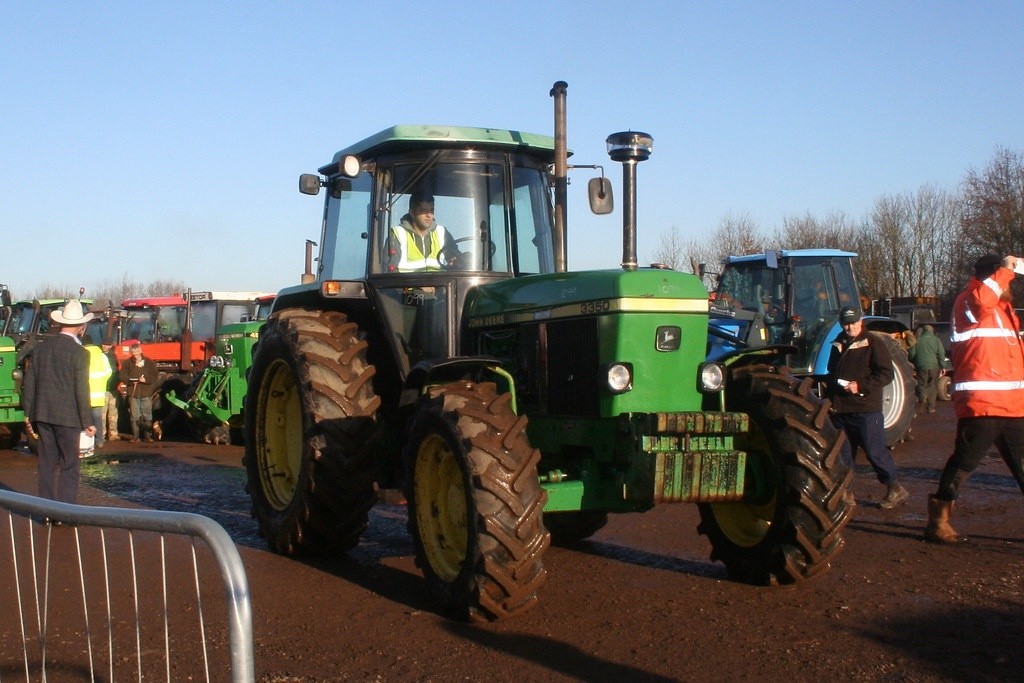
[703,243,917,451]
[239,76,855,628]
[0,283,276,445]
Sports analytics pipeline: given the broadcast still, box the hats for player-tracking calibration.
[975,254,1006,281]
[49,300,94,325]
[102,337,114,345]
[840,306,862,324]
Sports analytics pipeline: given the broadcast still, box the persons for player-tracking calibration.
[925,254,1024,542]
[22,299,96,527]
[384,191,464,300]
[120,344,160,444]
[76,334,112,450]
[826,308,910,508]
[94,336,121,441]
[911,326,947,413]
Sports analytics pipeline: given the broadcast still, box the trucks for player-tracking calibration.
[868,296,1023,403]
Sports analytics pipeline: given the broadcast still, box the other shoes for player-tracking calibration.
[146,437,154,443]
[108,435,120,442]
[927,408,936,413]
[95,441,104,448]
[129,437,141,443]
[918,406,924,413]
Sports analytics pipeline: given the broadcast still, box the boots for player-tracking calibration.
[925,494,968,546]
[880,481,909,510]
